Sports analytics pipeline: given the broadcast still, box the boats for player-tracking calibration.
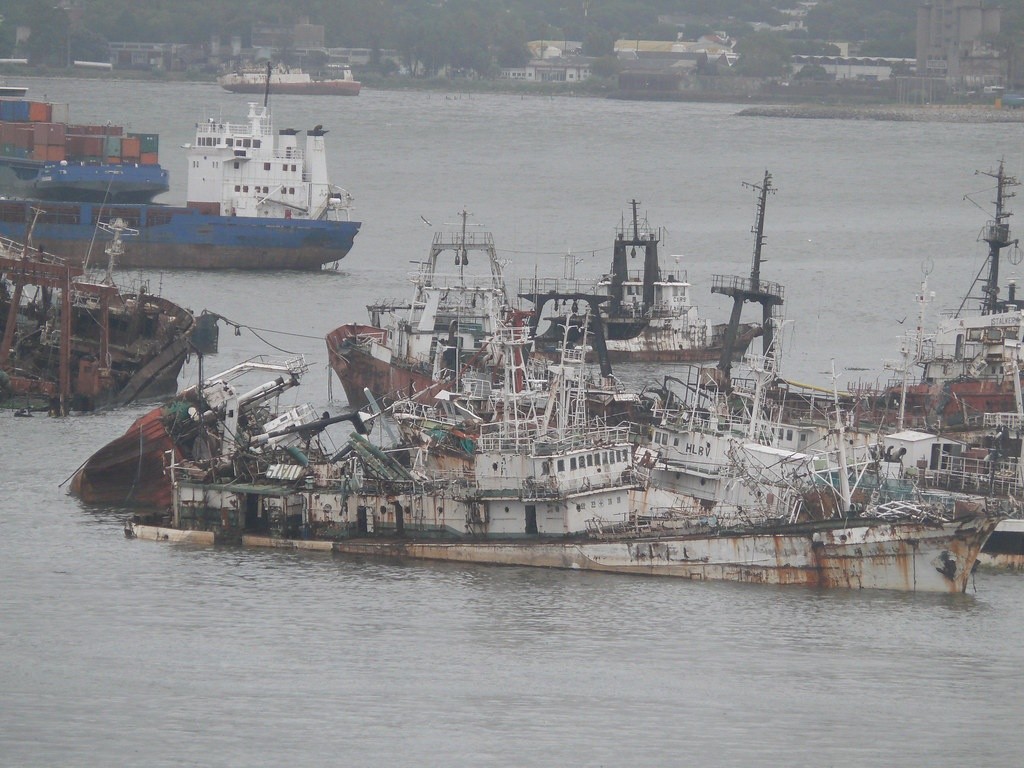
[733,154,1023,429]
[512,171,783,411]
[0,101,361,272]
[0,215,220,411]
[216,56,362,96]
[121,315,1016,595]
[325,324,1024,544]
[0,86,170,203]
